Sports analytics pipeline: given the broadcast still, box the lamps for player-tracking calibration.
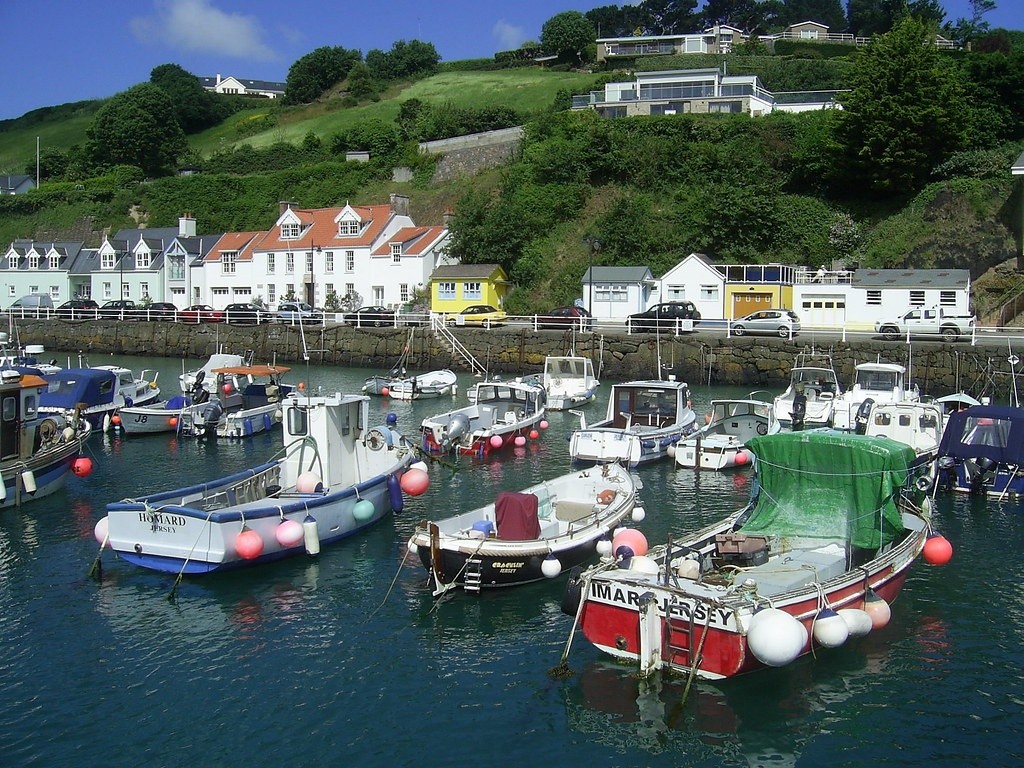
[516,377,524,384]
[669,375,676,382]
[49,358,57,365]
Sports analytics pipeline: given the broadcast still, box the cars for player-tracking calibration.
[446,305,507,327]
[5,293,323,325]
[344,306,397,327]
[730,309,801,338]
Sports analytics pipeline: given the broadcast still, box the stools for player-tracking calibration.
[474,521,493,537]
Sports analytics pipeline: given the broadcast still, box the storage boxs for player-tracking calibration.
[538,521,559,538]
[715,534,846,595]
[706,435,738,442]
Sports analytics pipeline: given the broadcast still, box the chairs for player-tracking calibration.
[505,411,517,423]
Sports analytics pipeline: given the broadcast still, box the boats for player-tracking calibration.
[106,393,416,573]
[466,356,599,409]
[411,462,645,594]
[27,365,161,433]
[0,367,92,508]
[577,426,931,681]
[193,365,297,436]
[116,353,254,435]
[0,332,62,375]
[418,377,546,455]
[569,352,1024,505]
[388,369,458,400]
[364,326,416,394]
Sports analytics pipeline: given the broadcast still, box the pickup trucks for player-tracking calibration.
[874,304,977,342]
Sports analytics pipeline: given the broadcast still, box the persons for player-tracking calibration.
[837,267,849,283]
[74,292,88,300]
[811,265,829,283]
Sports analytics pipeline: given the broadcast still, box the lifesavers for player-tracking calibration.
[561,566,584,618]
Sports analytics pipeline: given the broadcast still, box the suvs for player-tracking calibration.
[531,305,593,330]
[625,301,701,335]
